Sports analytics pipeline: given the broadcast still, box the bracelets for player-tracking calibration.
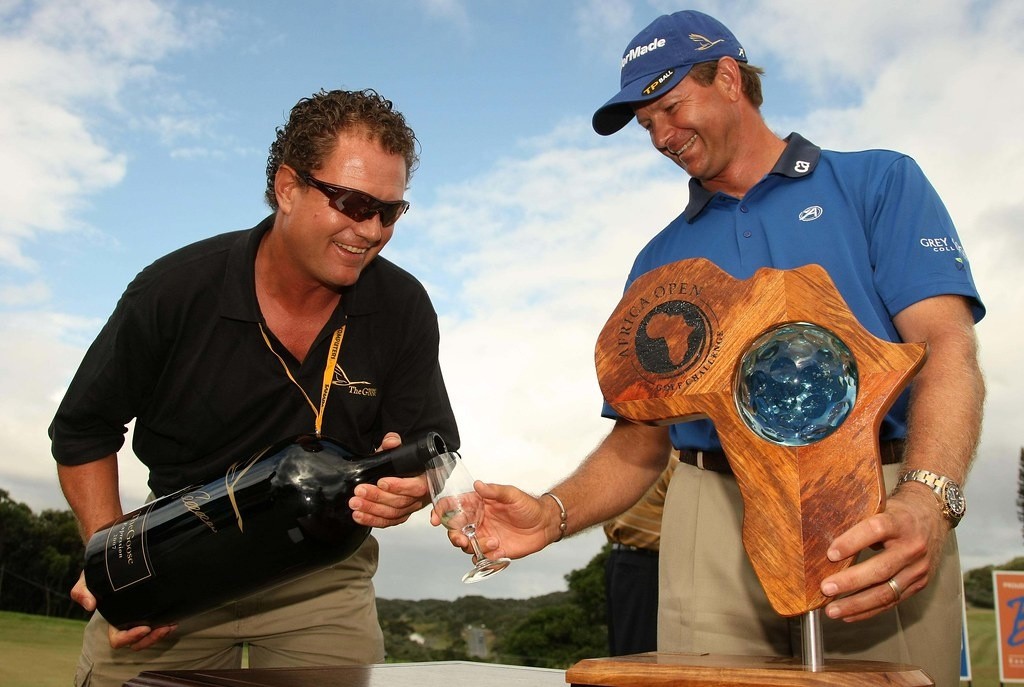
[541,490,570,547]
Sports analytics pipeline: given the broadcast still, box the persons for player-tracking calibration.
[48,86,459,687]
[431,9,985,687]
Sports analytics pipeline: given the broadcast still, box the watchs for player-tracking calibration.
[893,464,967,529]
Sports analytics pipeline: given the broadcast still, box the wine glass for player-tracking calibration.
[424,451,511,583]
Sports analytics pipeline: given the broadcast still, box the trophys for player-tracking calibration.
[596,258,929,618]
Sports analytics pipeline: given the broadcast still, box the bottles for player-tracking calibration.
[83,432,445,631]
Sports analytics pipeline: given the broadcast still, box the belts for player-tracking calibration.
[678,441,906,473]
[612,543,657,555]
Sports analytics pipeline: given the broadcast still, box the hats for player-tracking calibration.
[592,9,748,135]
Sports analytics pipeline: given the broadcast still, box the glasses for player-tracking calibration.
[290,162,411,227]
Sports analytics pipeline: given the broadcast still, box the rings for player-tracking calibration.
[887,577,903,601]
